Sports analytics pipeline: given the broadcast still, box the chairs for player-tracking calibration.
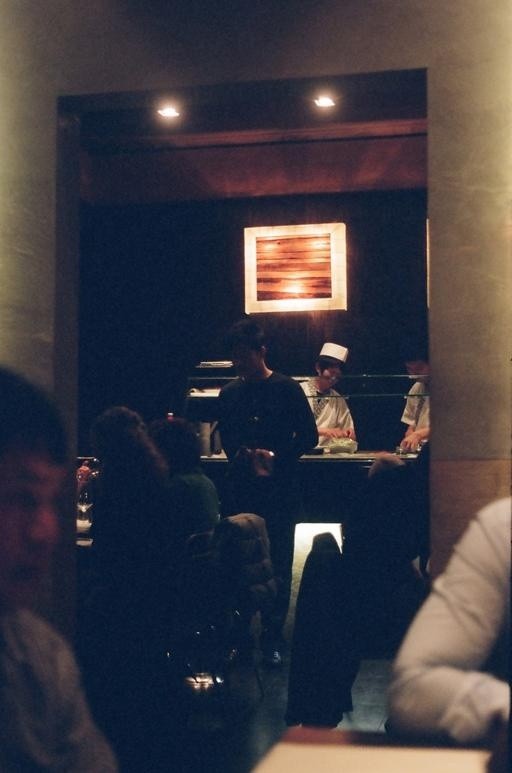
[132,513,285,772]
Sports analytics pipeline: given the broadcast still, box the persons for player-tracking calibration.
[1,362,128,771]
[365,442,432,556]
[210,316,322,673]
[380,496,511,773]
[82,403,222,575]
[297,340,359,449]
[395,337,431,456]
[75,459,101,506]
[148,415,237,531]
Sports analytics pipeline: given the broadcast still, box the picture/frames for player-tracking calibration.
[243,222,348,314]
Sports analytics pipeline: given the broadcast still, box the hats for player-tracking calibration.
[319,342,349,363]
